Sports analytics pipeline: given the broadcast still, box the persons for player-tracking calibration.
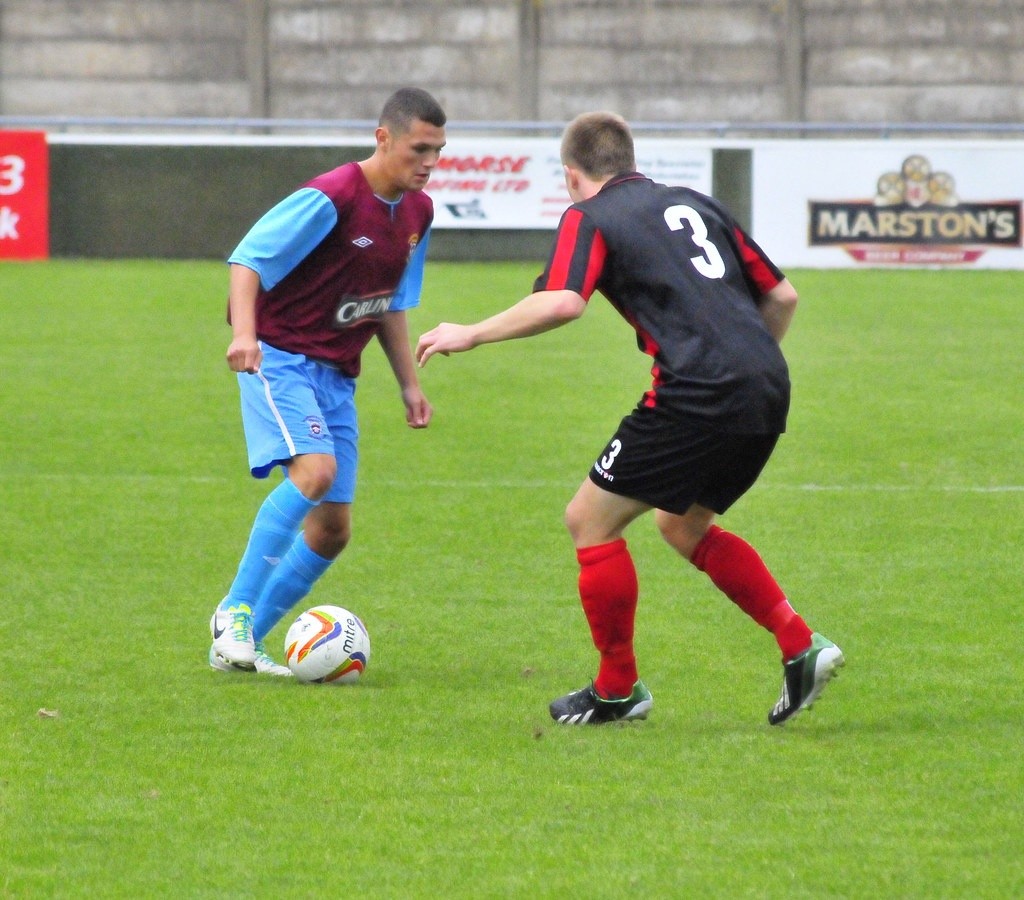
[416,112,846,724]
[207,86,432,677]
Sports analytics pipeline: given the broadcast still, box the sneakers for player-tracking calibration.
[768,632,848,725]
[209,641,293,677]
[549,676,654,725]
[210,595,258,663]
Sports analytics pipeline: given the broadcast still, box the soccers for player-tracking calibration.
[283,603,371,686]
